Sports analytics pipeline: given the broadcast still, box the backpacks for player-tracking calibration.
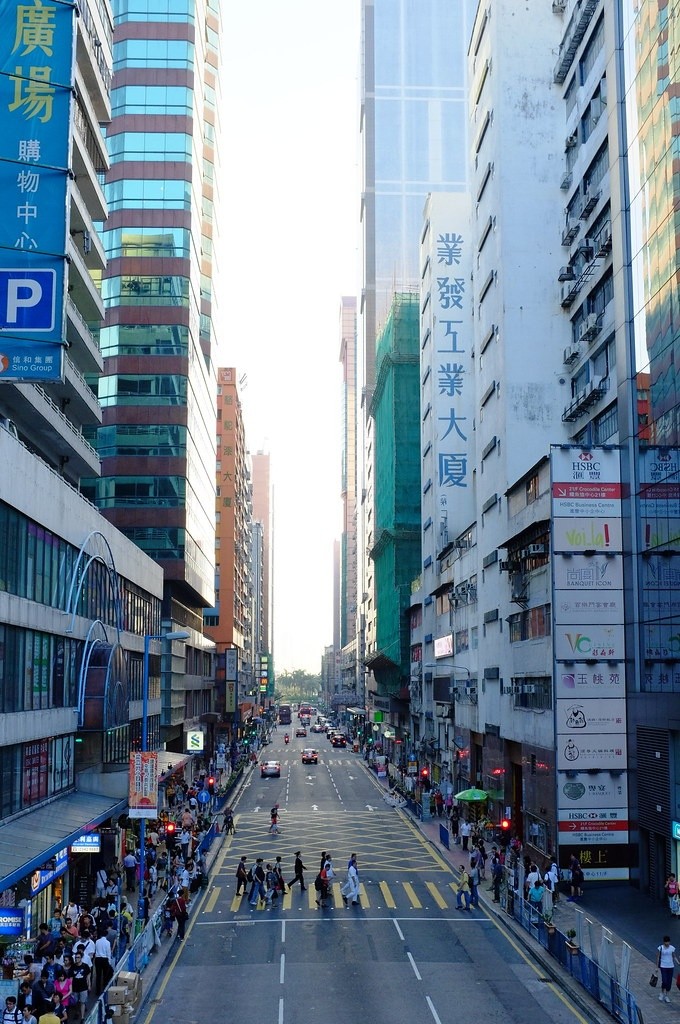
[97,907,109,926]
[255,865,266,882]
[315,872,324,891]
[464,872,473,888]
[553,865,564,881]
[247,864,256,883]
[113,914,125,937]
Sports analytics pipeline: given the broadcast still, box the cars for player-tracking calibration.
[296,727,307,738]
[297,703,317,726]
[310,725,324,733]
[317,714,346,748]
[300,748,319,764]
[260,760,281,778]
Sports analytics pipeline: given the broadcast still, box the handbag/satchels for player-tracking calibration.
[672,894,680,916]
[542,872,552,890]
[180,910,190,921]
[649,971,658,988]
[482,854,488,860]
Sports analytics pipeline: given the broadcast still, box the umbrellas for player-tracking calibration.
[455,786,487,822]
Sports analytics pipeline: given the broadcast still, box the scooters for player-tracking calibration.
[285,735,289,744]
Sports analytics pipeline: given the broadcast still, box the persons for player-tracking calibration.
[287,851,307,891]
[362,737,406,779]
[450,809,488,851]
[469,840,488,881]
[220,806,236,836]
[0,856,134,1024]
[268,804,282,835]
[665,872,680,912]
[456,862,479,910]
[490,835,560,914]
[567,854,585,903]
[655,935,680,1002]
[285,733,289,742]
[137,887,194,942]
[335,717,340,729]
[221,729,260,775]
[235,856,288,908]
[124,765,225,908]
[340,853,360,906]
[315,852,337,910]
[422,776,454,820]
[274,723,276,729]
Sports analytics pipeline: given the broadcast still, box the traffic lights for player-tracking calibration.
[208,777,214,795]
[422,770,427,785]
[165,822,175,850]
[501,819,511,846]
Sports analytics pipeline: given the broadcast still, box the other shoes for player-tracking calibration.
[168,930,173,937]
[272,906,279,908]
[554,904,557,907]
[250,901,257,906]
[276,830,281,834]
[473,904,478,906]
[321,904,329,908]
[302,888,307,890]
[554,900,561,903]
[185,904,189,908]
[315,900,321,906]
[455,905,462,909]
[482,876,487,881]
[492,899,500,903]
[243,891,250,894]
[236,893,241,896]
[282,892,288,895]
[463,907,470,911]
[178,935,184,942]
[486,887,492,891]
[234,832,237,834]
[268,829,271,833]
[260,900,263,906]
[220,830,223,832]
[287,884,291,889]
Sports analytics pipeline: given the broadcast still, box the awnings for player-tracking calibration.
[347,707,366,715]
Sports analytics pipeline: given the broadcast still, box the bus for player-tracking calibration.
[278,703,292,725]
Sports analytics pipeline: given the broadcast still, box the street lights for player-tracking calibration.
[136,631,191,918]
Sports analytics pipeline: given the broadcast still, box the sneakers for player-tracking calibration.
[659,993,671,1003]
[566,896,580,902]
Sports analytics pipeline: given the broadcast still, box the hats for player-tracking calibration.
[294,851,300,855]
